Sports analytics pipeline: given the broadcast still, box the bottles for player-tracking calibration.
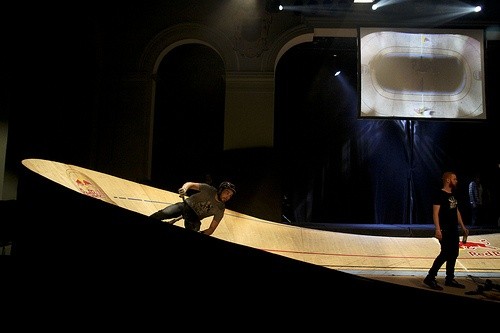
[463,233,468,244]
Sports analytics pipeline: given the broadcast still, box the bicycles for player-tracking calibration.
[165,188,200,233]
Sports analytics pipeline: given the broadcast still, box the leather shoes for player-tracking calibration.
[424,276,443,290]
[445,280,465,288]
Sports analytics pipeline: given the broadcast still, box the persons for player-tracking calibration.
[468,175,480,226]
[424,172,468,291]
[148,181,236,234]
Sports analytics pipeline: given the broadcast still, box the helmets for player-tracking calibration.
[219,182,236,195]
